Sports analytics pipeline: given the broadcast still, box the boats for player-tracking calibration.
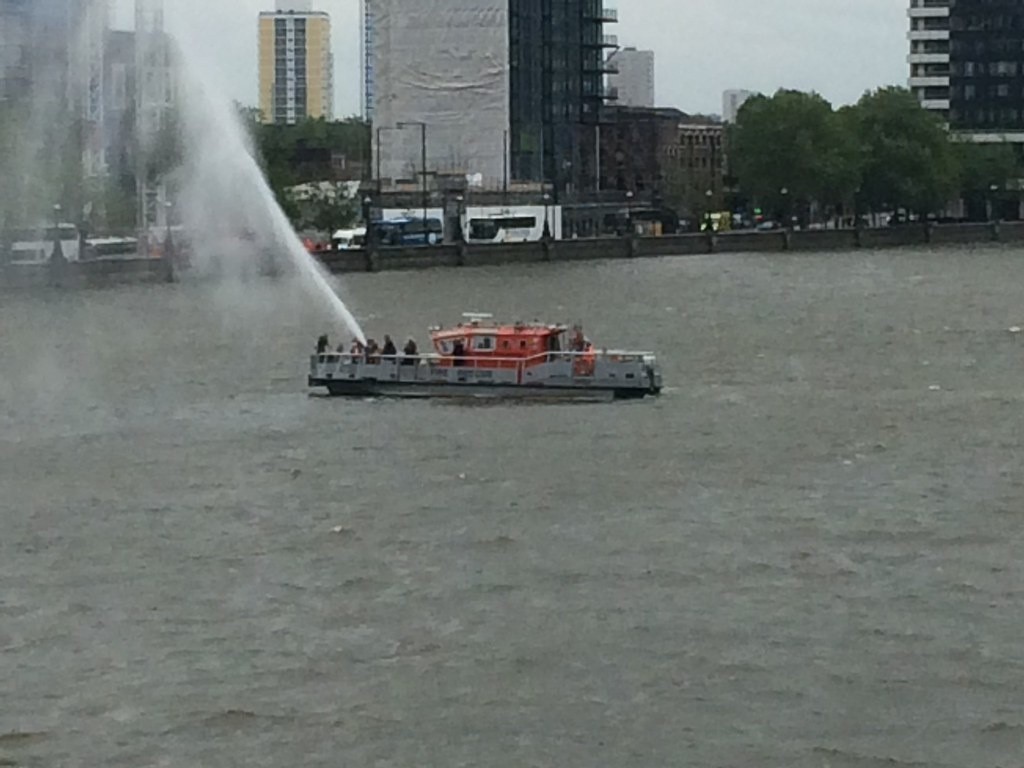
[306,312,664,404]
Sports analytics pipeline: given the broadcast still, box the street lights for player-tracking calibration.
[51,203,65,259]
[989,185,1000,223]
[165,201,174,254]
[626,191,634,235]
[541,191,551,238]
[455,194,465,241]
[704,188,714,233]
[779,186,792,228]
[363,194,374,244]
[373,125,405,220]
[397,121,429,244]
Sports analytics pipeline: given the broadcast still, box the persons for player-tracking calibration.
[317,333,343,377]
[451,339,466,381]
[402,340,417,365]
[349,337,378,378]
[569,324,595,362]
[478,338,491,349]
[381,335,396,363]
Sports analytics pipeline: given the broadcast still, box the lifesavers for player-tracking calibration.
[573,356,595,376]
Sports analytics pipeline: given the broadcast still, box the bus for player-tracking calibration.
[360,215,445,247]
[466,211,540,245]
[7,222,80,266]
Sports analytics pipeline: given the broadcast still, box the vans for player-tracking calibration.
[326,227,365,251]
